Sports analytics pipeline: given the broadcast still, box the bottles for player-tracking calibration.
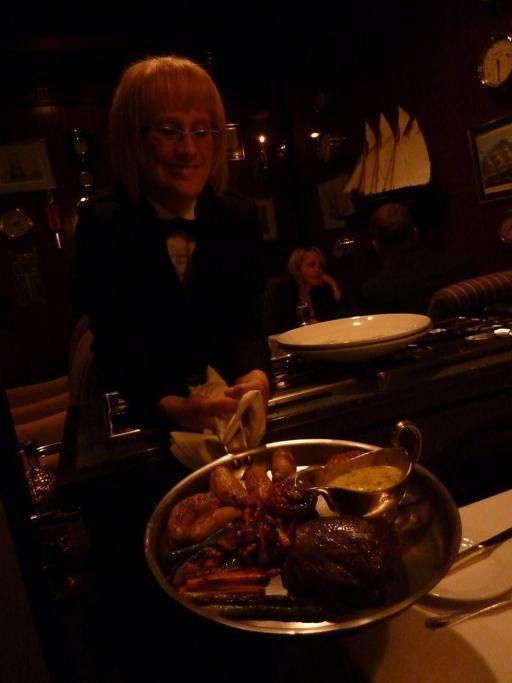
[21,440,54,502]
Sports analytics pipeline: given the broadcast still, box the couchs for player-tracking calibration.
[429,269,512,318]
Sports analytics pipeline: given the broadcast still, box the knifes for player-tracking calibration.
[450,526,511,565]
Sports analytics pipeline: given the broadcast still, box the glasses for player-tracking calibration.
[155,125,220,147]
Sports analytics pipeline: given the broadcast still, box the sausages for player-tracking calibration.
[167,448,298,549]
[180,572,266,600]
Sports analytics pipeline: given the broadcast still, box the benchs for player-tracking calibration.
[6,374,73,449]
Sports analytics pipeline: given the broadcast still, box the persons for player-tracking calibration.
[50,56,279,682]
[362,201,457,313]
[285,244,343,329]
[482,137,512,177]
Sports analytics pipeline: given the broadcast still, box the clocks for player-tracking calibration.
[473,28,512,91]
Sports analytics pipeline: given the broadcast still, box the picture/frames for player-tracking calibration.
[465,111,512,204]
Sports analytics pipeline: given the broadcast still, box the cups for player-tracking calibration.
[292,420,423,516]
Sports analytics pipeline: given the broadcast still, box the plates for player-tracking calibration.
[274,312,437,360]
[416,512,512,605]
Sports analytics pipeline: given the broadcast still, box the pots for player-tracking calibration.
[142,379,465,639]
